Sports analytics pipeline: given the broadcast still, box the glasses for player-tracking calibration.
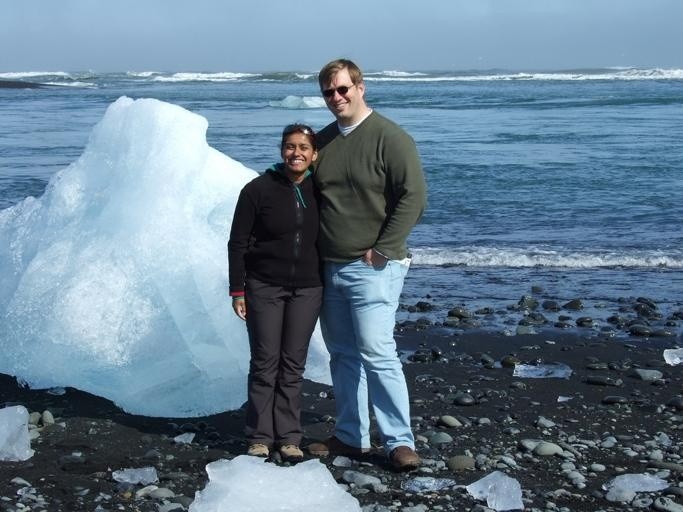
[321,83,358,97]
[283,125,313,135]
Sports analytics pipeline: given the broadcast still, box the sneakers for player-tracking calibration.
[309,435,373,456]
[388,445,420,471]
[247,444,270,458]
[279,442,304,462]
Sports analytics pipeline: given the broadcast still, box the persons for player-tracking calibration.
[226,122,323,463]
[309,58,426,471]
